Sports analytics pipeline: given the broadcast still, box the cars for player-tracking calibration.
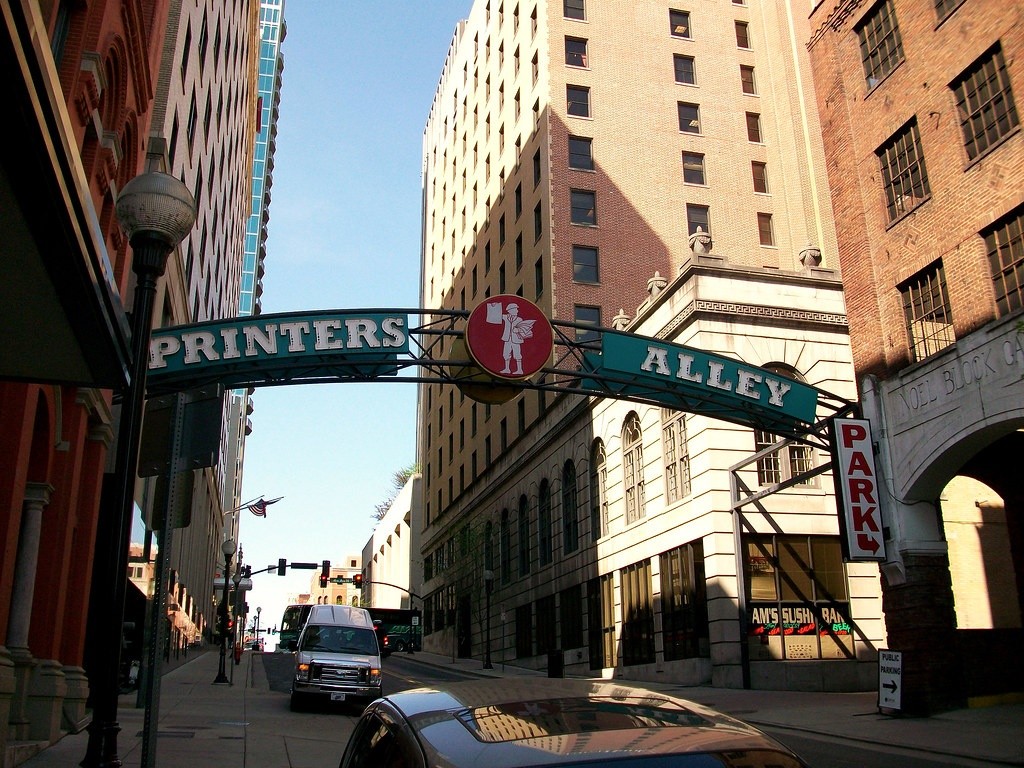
[338,677,812,768]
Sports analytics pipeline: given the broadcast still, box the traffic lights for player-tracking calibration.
[268,628,270,634]
[320,574,327,588]
[278,558,287,576]
[355,574,362,589]
[272,629,275,635]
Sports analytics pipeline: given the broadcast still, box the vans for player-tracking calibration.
[371,620,391,658]
[289,604,383,710]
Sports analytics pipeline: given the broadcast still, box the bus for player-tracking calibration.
[279,604,422,651]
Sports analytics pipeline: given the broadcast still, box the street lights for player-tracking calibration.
[211,539,236,684]
[78,169,198,768]
[256,607,261,644]
[481,569,495,669]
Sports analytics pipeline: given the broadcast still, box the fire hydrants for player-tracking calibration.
[234,643,241,666]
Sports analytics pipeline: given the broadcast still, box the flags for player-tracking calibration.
[247,498,279,518]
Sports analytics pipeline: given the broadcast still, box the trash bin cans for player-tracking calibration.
[546,649,565,678]
